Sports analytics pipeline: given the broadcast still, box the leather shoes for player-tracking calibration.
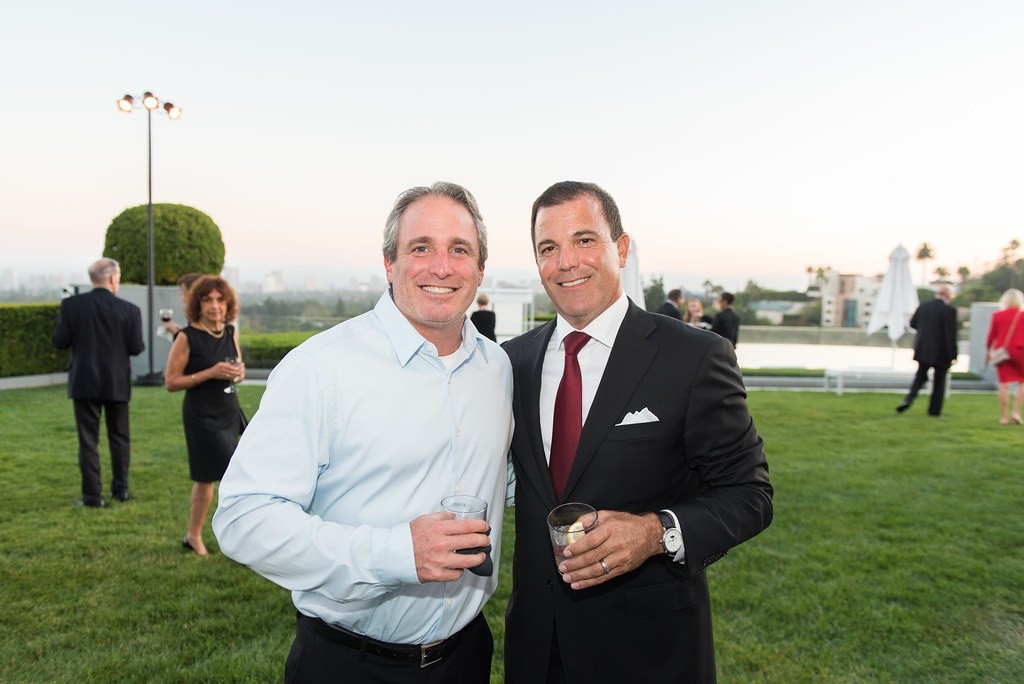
[83,493,107,509]
[109,489,131,502]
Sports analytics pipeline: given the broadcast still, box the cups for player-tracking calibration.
[547,503,598,576]
[441,494,488,523]
[159,309,173,324]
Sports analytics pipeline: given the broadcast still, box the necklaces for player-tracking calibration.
[199,319,224,339]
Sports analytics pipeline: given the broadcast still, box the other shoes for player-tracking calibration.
[183,536,206,558]
[1000,419,1008,425]
[1011,412,1024,426]
[897,400,909,412]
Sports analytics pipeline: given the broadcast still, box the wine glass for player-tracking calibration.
[224,356,240,394]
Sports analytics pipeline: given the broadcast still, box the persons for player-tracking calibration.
[895,285,958,418]
[711,293,739,350]
[683,298,713,330]
[164,273,202,334]
[471,295,496,343]
[53,257,143,509]
[654,290,683,320]
[166,275,245,557]
[211,184,517,684]
[985,289,1024,425]
[499,181,774,684]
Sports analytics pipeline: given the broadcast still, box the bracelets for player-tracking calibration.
[192,374,197,385]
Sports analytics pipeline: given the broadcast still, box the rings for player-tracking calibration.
[227,373,229,378]
[600,559,609,573]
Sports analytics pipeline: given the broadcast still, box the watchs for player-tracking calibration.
[655,512,682,557]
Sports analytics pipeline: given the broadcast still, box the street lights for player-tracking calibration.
[119,92,181,374]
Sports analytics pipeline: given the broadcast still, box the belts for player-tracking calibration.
[300,612,479,670]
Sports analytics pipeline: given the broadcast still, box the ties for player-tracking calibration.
[548,329,592,505]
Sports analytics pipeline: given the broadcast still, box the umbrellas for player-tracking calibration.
[866,244,919,347]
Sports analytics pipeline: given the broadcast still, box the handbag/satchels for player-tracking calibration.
[988,346,1009,365]
[237,408,249,434]
